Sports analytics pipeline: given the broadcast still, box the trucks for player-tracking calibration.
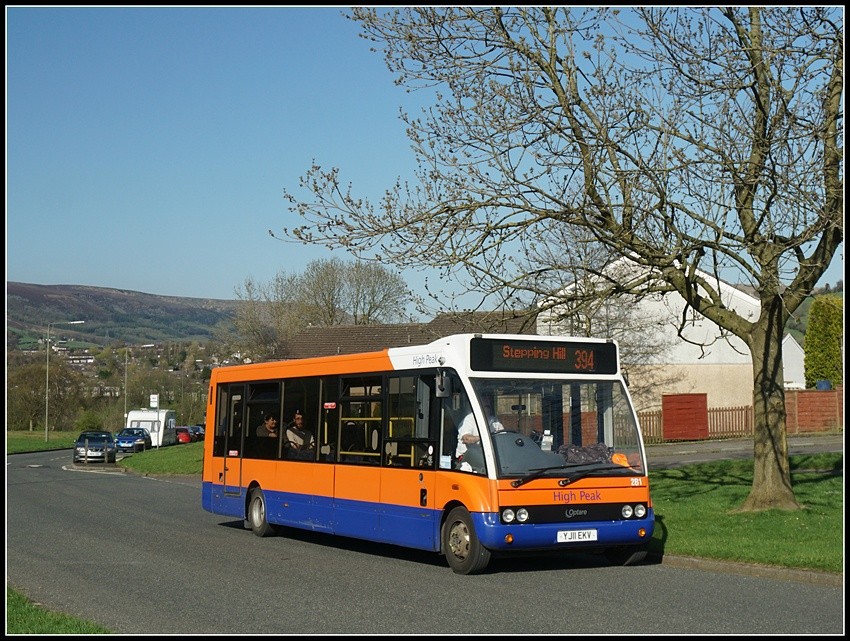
[124,408,175,447]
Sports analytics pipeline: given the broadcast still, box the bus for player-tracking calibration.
[201,333,655,574]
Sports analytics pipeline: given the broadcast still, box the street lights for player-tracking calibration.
[181,360,203,425]
[45,321,85,443]
[124,344,154,428]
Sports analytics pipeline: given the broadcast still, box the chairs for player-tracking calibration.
[342,421,381,460]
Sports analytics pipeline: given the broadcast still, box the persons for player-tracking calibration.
[456,393,505,457]
[286,409,315,450]
[256,412,277,437]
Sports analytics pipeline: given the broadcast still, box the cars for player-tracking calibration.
[175,424,205,444]
[73,430,117,463]
[114,427,152,453]
